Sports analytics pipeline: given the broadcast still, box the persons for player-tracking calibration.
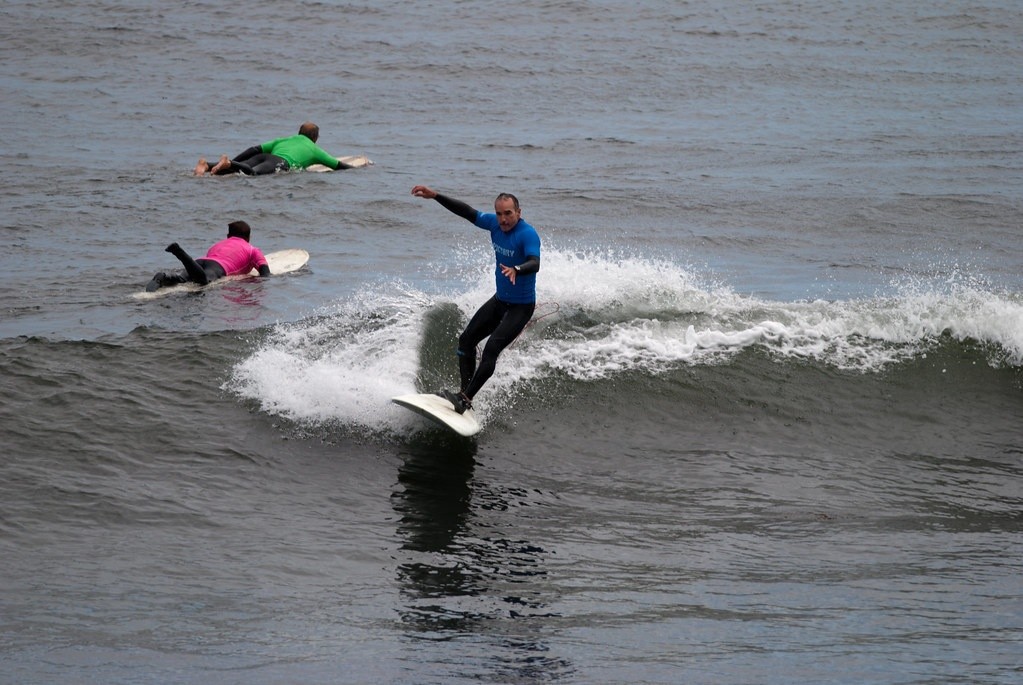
[193,123,353,177]
[147,220,272,291]
[411,185,541,415]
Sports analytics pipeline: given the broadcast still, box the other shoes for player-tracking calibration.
[439,391,445,397]
[444,388,468,415]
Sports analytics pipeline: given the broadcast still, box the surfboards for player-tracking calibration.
[390,392,484,438]
[303,155,369,173]
[128,248,311,300]
